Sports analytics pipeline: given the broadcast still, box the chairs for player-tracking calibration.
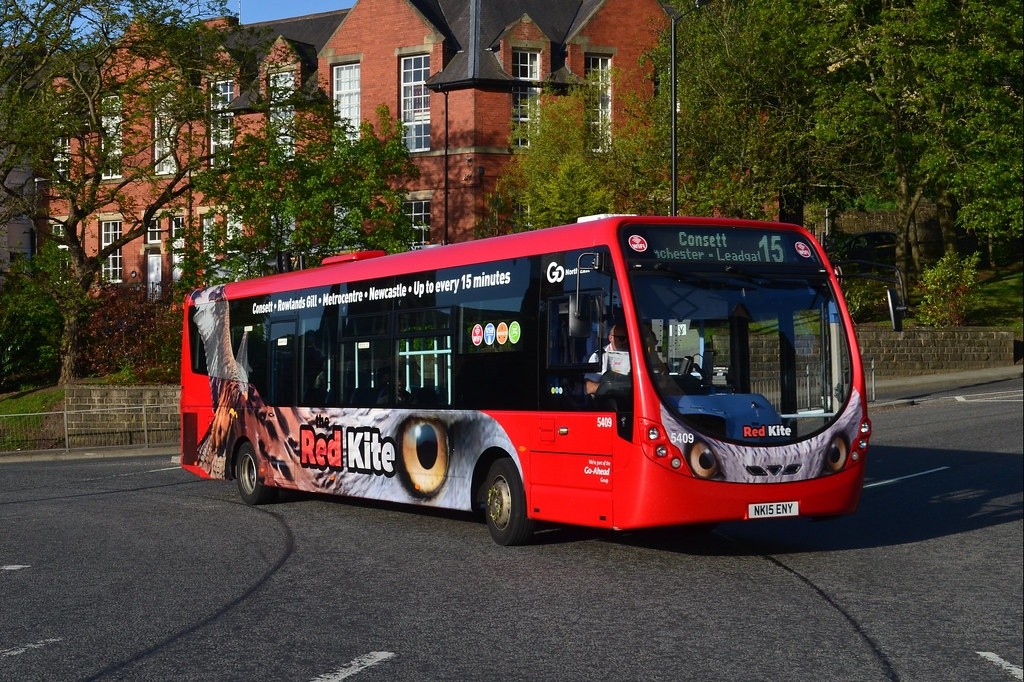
[573,322,612,410]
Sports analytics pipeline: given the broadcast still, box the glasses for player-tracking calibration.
[611,335,628,342]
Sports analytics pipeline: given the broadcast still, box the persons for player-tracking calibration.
[585,324,629,394]
[409,355,421,379]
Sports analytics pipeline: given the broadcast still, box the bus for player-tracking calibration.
[178,213,909,545]
